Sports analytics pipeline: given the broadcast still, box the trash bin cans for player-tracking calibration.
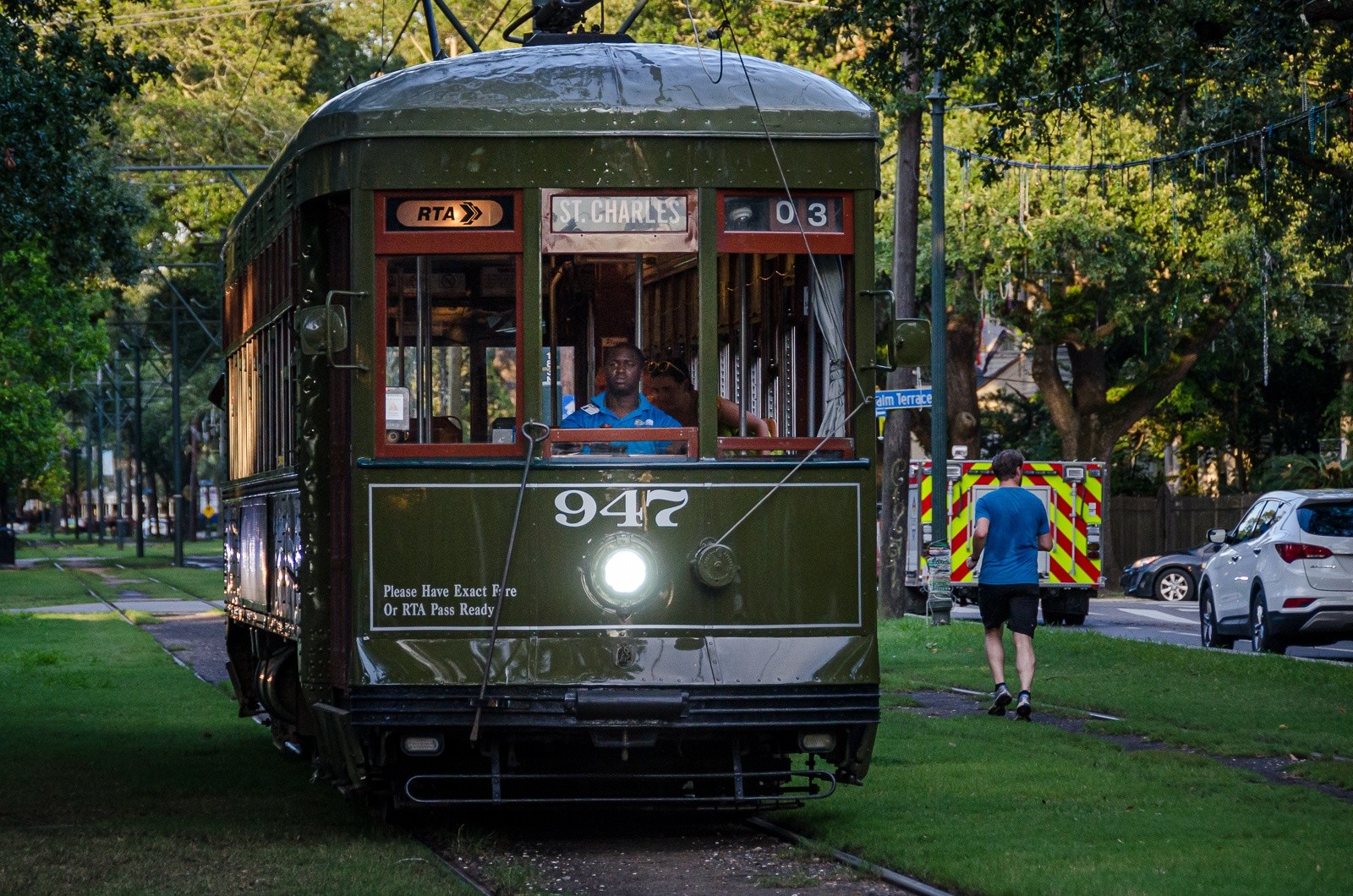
[0,527,15,564]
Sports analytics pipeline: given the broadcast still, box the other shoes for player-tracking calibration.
[1014,693,1032,721]
[987,682,1012,716]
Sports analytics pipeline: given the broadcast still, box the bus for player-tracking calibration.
[223,0,931,822]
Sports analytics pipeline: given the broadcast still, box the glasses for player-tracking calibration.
[647,360,686,378]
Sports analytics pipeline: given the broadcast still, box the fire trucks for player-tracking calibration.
[905,446,1105,627]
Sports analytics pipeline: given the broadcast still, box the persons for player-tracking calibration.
[647,358,785,458]
[552,341,687,455]
[965,448,1053,722]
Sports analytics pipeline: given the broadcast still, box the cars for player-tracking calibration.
[1119,529,1234,602]
[143,518,173,542]
[1198,488,1353,655]
